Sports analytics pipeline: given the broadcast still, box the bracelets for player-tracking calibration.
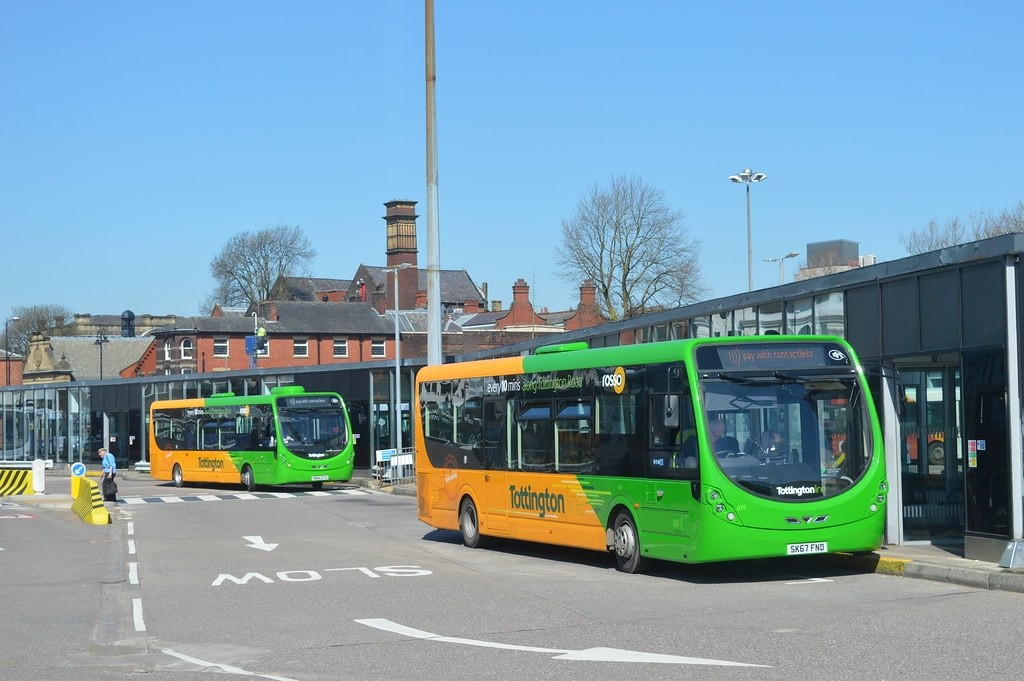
[110,473,113,474]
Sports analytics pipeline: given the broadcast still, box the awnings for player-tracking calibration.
[519,403,593,421]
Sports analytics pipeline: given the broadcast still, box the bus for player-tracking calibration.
[414,335,887,574]
[829,375,960,466]
[149,386,355,491]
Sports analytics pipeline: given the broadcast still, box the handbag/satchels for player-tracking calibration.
[105,478,118,496]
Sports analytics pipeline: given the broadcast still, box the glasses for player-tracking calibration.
[712,430,724,439]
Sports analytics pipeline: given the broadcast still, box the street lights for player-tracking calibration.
[381,263,411,480]
[5,317,20,385]
[763,252,800,285]
[729,168,767,292]
[93,328,110,380]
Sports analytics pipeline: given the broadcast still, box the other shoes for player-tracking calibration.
[104,497,117,502]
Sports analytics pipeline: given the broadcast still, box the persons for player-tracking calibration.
[98,448,118,502]
[676,418,739,458]
[269,424,295,447]
